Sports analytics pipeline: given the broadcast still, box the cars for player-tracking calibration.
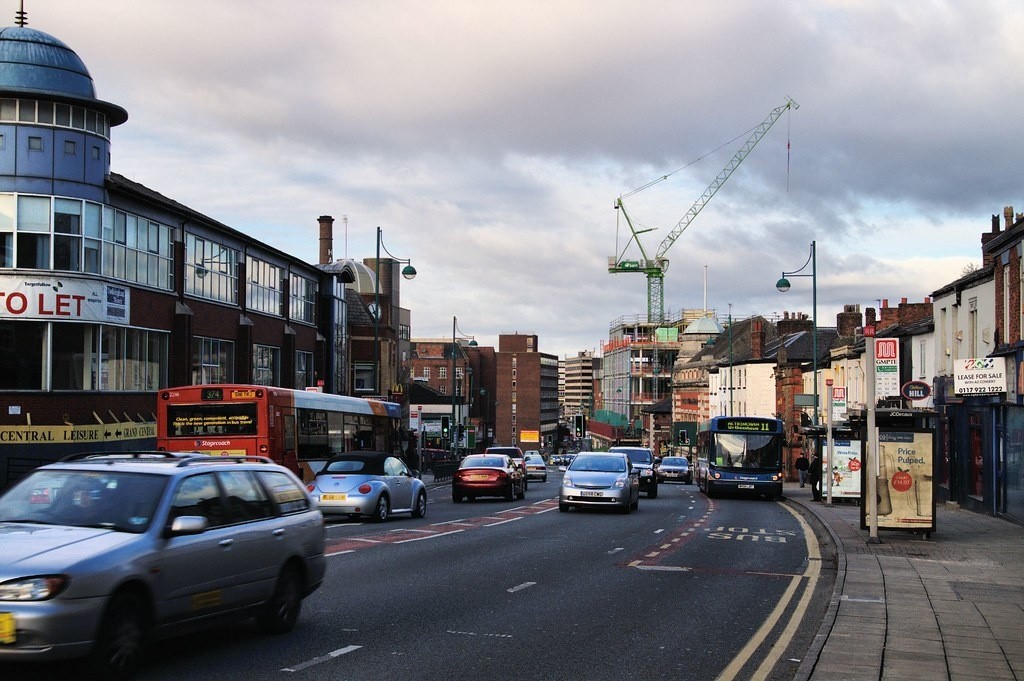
[549,453,575,467]
[452,454,525,501]
[524,450,548,482]
[304,450,429,522]
[657,456,694,485]
[558,451,642,514]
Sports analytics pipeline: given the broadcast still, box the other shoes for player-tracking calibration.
[810,499,819,502]
[801,485,805,488]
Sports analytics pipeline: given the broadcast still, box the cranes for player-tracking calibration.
[608,94,800,324]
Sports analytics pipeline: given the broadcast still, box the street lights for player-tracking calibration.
[487,389,500,443]
[469,376,486,425]
[450,316,478,447]
[776,240,821,452]
[654,352,672,442]
[704,314,734,417]
[372,226,416,393]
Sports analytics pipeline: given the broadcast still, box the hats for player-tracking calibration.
[812,453,818,456]
[799,452,805,454]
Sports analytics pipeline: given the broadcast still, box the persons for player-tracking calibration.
[539,446,588,465]
[401,451,461,472]
[808,452,821,502]
[796,453,809,488]
[686,453,692,463]
[653,452,667,469]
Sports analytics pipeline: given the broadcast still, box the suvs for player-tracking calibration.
[607,447,662,499]
[0,449,327,681]
[484,444,530,491]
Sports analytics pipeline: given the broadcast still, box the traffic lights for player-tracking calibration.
[456,422,465,442]
[425,431,429,441]
[427,442,431,446]
[678,430,690,446]
[422,431,425,440]
[575,415,583,439]
[441,415,450,441]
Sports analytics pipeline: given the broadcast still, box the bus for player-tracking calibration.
[156,386,402,486]
[693,416,788,502]
